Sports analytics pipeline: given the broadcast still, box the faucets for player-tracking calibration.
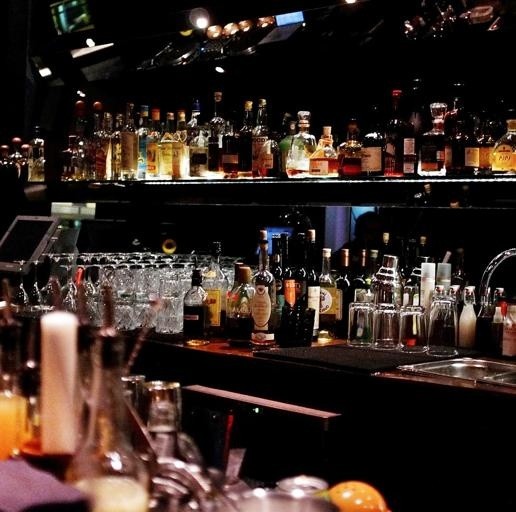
[478,246,516,297]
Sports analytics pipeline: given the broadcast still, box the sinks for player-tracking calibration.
[492,371,516,385]
[412,359,512,380]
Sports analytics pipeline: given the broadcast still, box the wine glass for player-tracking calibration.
[10,251,245,337]
[0,301,55,428]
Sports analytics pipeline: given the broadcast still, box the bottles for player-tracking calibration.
[63,324,239,512]
[456,285,516,357]
[351,230,468,306]
[184,227,350,355]
[0,90,514,182]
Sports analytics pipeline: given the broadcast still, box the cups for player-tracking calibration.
[397,305,428,355]
[427,300,459,359]
[369,301,400,351]
[346,301,379,349]
